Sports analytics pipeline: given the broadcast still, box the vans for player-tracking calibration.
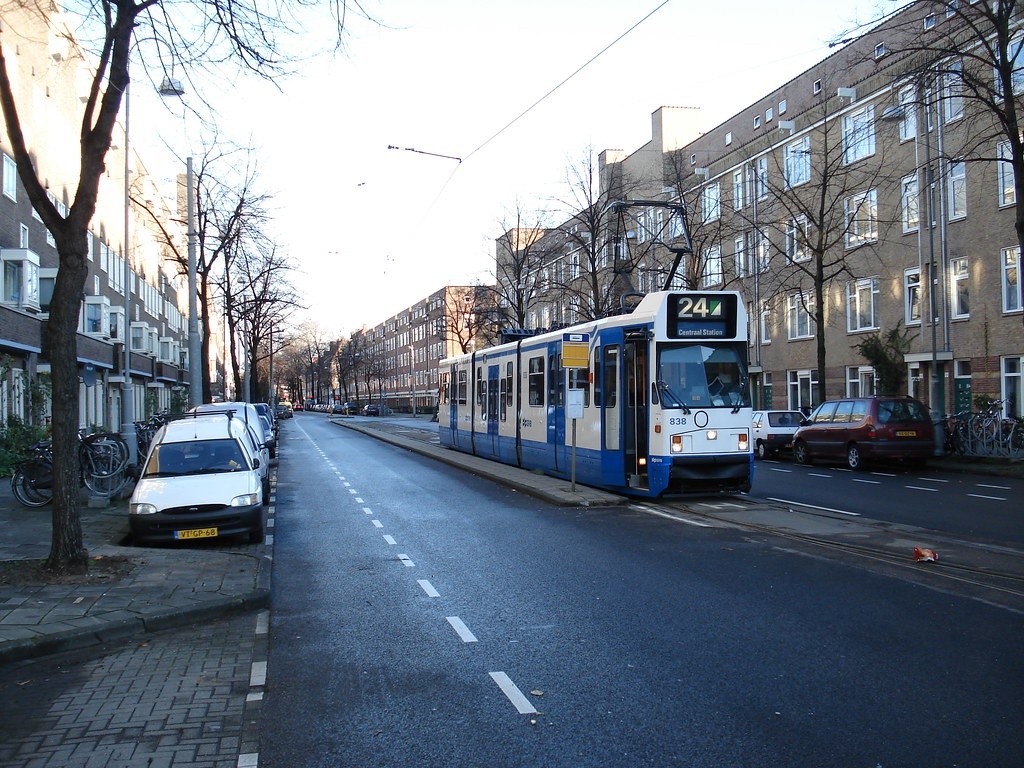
[184,401,277,507]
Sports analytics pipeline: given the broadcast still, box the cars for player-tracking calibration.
[362,405,379,417]
[275,399,358,420]
[251,402,279,459]
[129,409,268,545]
[752,409,810,459]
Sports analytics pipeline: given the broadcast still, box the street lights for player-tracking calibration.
[880,71,948,460]
[626,209,656,294]
[122,36,185,501]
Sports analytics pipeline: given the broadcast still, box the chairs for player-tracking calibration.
[164,451,200,471]
[215,446,235,462]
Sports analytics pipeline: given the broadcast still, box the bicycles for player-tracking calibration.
[933,399,1024,458]
[10,428,132,509]
[133,407,168,467]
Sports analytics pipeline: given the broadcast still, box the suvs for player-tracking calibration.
[791,395,935,476]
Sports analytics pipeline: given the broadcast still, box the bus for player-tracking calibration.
[438,200,753,501]
